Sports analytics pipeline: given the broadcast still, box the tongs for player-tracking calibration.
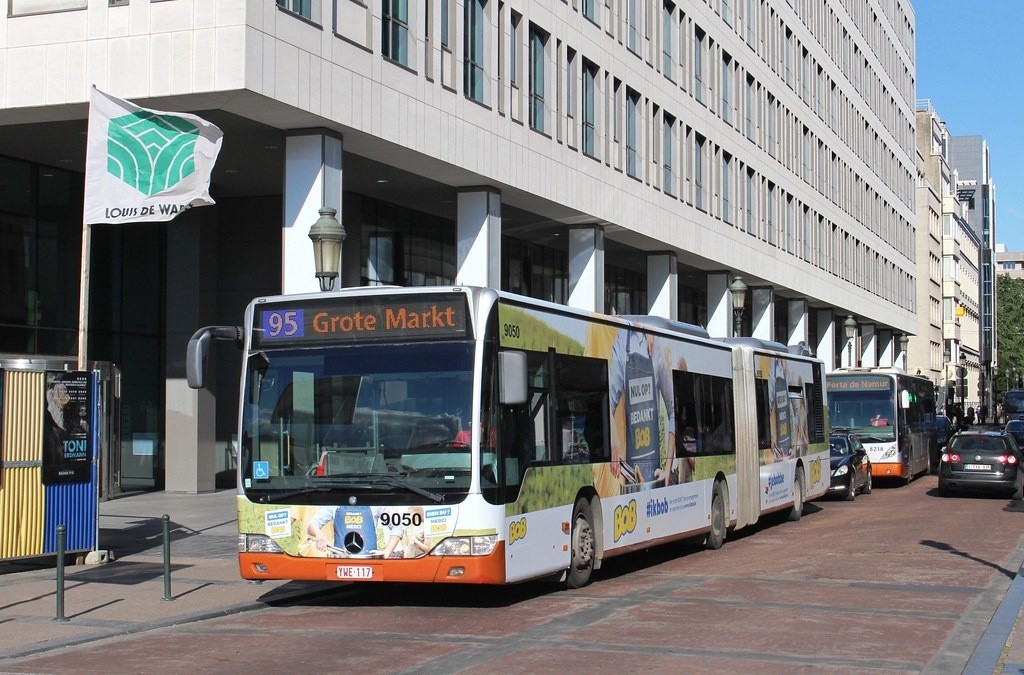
[619,458,636,485]
[309,539,345,556]
[774,444,782,457]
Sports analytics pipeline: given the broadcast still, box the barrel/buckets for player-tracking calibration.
[774,455,794,463]
[620,479,665,495]
[327,553,383,559]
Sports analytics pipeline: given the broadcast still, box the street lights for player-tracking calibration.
[944,347,950,412]
[844,312,856,366]
[308,205,347,462]
[1006,367,1011,393]
[979,359,987,423]
[959,353,966,415]
[728,275,748,338]
[900,331,910,373]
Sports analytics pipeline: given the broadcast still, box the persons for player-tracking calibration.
[768,355,809,461]
[869,405,889,427]
[831,440,846,456]
[452,418,496,447]
[307,506,433,558]
[608,319,697,489]
[966,403,1003,425]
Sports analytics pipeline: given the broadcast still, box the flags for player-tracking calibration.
[85,85,225,228]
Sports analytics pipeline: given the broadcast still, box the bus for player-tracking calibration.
[826,365,937,486]
[1005,388,1024,424]
[186,285,830,589]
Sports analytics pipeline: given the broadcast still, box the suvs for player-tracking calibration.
[817,428,872,501]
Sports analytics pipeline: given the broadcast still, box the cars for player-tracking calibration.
[936,415,1024,500]
